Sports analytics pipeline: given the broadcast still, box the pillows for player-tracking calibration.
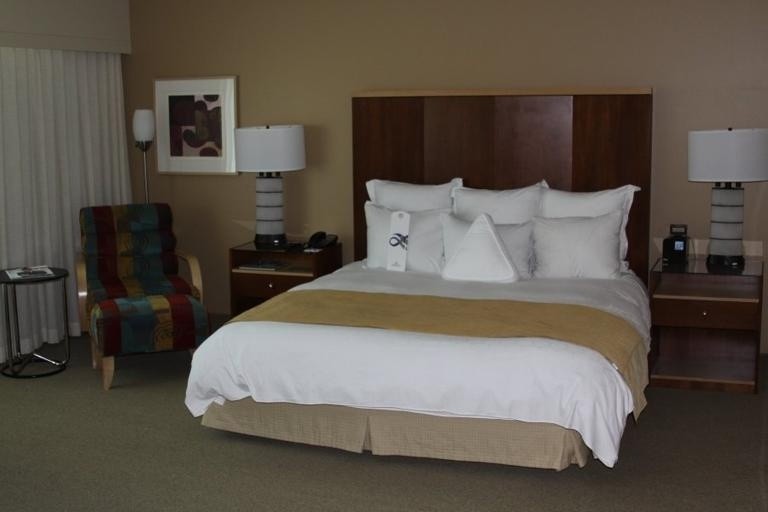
[361,173,642,284]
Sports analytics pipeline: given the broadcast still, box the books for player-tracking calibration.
[238,259,297,271]
[4,265,56,282]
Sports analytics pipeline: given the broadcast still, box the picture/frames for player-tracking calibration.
[152,73,240,178]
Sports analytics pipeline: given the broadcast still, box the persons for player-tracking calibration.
[17,266,47,276]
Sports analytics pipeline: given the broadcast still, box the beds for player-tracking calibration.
[184,87,655,473]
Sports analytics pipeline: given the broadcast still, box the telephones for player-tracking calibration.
[308,231,338,249]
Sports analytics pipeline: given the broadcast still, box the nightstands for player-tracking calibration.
[227,231,344,320]
[645,251,763,396]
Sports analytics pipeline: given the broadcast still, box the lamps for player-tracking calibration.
[231,123,307,249]
[132,107,157,204]
[687,126,768,267]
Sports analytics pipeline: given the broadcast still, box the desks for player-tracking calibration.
[0,266,73,378]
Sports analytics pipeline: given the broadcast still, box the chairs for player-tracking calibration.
[71,201,205,393]
